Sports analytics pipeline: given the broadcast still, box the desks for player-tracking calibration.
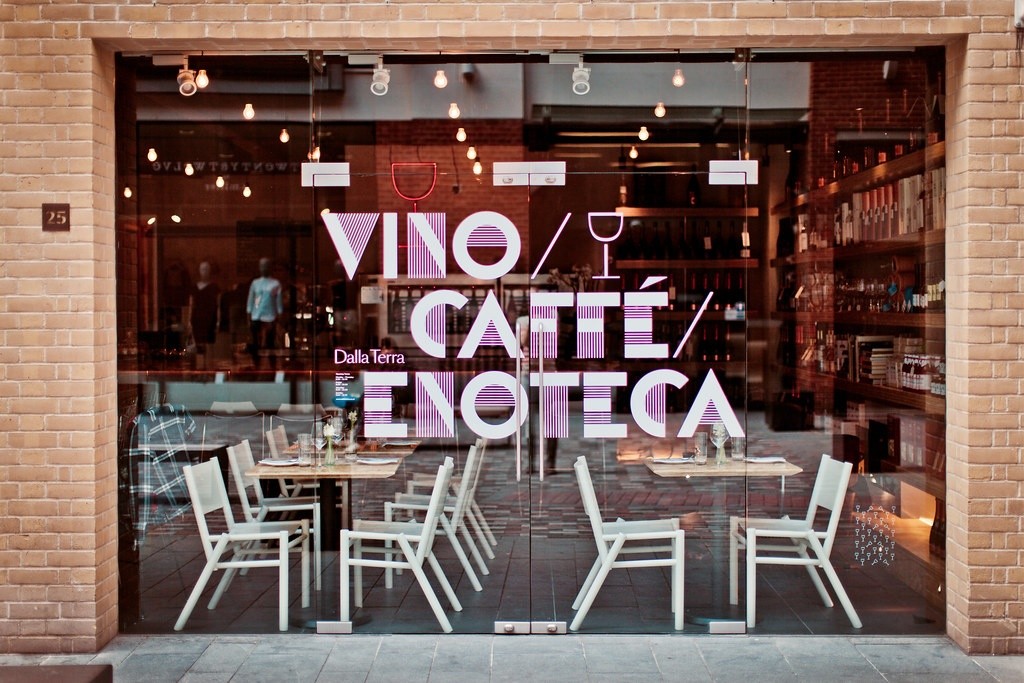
[245,440,420,627]
[644,458,803,626]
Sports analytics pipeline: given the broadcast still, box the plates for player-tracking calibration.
[743,456,786,463]
[653,456,694,463]
[357,457,400,464]
[258,457,299,466]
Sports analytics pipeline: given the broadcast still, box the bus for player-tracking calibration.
[358,273,588,368]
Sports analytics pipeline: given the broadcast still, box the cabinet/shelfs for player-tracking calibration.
[612,204,760,372]
[775,138,945,500]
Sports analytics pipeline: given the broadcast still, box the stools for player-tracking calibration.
[200,400,265,464]
[268,404,332,461]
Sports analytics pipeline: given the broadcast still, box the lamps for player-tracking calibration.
[177,60,594,103]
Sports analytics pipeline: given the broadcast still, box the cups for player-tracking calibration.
[731,437,744,461]
[298,433,312,466]
[694,432,708,464]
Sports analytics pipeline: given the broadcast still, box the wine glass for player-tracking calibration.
[709,422,730,465]
[328,417,344,465]
[833,273,888,312]
[311,422,328,470]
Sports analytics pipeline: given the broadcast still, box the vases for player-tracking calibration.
[325,435,335,467]
[346,429,357,453]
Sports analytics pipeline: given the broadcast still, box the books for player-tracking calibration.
[835,166,945,246]
[859,346,894,386]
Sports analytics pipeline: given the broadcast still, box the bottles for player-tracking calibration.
[902,354,946,397]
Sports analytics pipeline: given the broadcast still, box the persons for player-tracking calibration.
[188,262,222,370]
[352,337,411,447]
[245,257,284,370]
[513,290,558,474]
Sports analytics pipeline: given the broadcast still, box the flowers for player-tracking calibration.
[323,424,334,436]
[349,410,357,430]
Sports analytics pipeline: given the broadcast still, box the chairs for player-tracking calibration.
[265,426,349,546]
[730,453,863,628]
[569,454,685,630]
[183,439,498,632]
